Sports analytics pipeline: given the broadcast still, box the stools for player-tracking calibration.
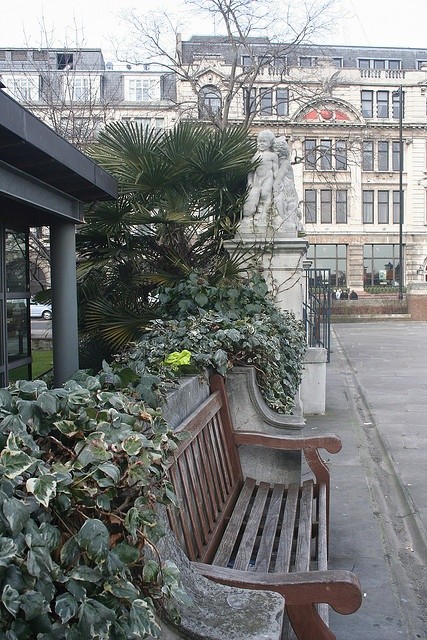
[146,370,363,639]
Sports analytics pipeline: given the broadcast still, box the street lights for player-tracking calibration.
[391,84,404,299]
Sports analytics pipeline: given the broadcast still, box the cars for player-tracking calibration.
[27,296,52,320]
